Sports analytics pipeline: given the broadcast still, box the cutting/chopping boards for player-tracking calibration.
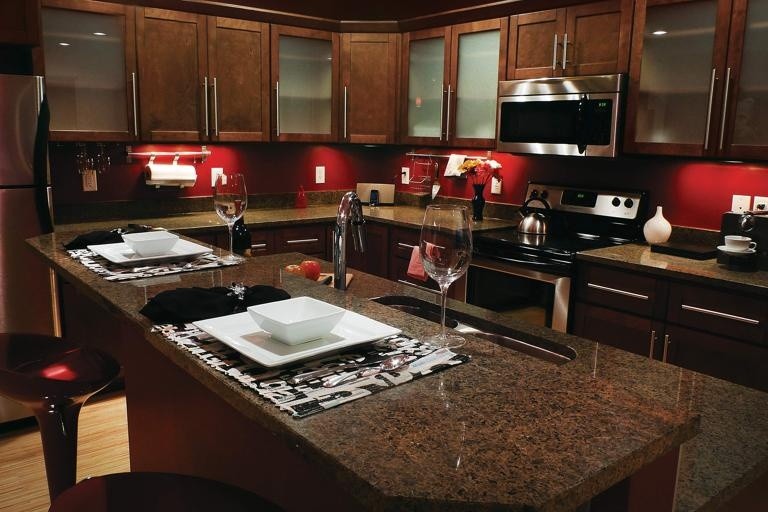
[314,273,353,289]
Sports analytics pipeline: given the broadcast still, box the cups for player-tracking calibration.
[370,189,379,207]
[725,236,758,250]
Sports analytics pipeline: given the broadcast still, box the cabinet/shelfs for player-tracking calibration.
[402,16,509,148]
[622,0,768,160]
[566,261,768,393]
[339,33,402,145]
[39,0,139,141]
[506,0,634,80]
[389,226,466,303]
[216,225,326,261]
[136,6,271,143]
[270,23,340,143]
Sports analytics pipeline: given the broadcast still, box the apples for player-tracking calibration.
[302,260,321,281]
[284,264,306,278]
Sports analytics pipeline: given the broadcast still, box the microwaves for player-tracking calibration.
[497,75,625,159]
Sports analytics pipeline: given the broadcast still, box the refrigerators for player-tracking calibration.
[0,70,61,431]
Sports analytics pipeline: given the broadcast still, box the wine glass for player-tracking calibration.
[213,173,249,263]
[73,144,111,176]
[418,203,474,350]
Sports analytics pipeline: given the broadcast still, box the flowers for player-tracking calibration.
[457,160,503,185]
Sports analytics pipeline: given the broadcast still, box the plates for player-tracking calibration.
[718,246,755,257]
[192,296,403,369]
[87,238,213,267]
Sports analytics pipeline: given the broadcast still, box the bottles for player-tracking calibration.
[296,185,308,209]
[644,204,673,245]
[230,200,251,258]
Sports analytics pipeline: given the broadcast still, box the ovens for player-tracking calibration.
[452,254,574,334]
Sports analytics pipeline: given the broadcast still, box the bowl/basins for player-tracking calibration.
[122,231,178,256]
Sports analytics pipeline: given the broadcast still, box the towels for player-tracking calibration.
[407,246,429,282]
[425,242,441,261]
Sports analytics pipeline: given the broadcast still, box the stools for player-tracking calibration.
[48,471,285,512]
[1,332,121,507]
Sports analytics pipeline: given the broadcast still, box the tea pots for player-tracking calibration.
[515,198,552,236]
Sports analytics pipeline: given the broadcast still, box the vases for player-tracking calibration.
[472,184,485,220]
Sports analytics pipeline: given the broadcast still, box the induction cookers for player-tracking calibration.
[474,223,642,268]
